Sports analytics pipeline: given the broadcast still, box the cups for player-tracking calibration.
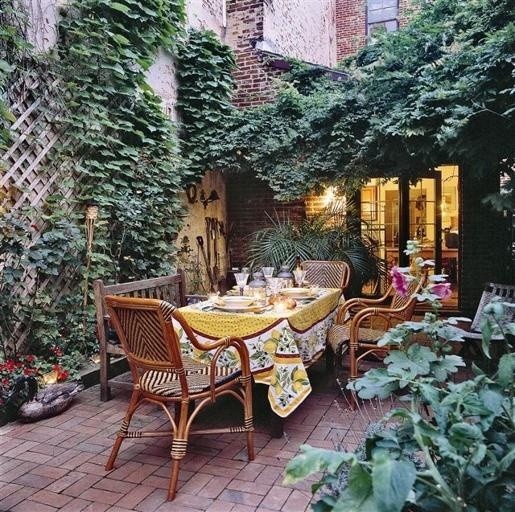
[207,293,218,305]
[292,270,307,287]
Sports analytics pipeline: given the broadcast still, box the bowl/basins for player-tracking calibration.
[223,297,253,306]
[283,288,309,297]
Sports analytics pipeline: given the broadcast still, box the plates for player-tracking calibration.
[212,302,258,312]
[288,296,316,299]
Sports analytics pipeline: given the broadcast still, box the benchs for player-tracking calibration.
[93,268,207,411]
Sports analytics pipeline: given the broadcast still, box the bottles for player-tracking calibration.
[278,255,303,287]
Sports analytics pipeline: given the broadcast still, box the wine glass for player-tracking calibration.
[234,273,248,296]
[262,267,282,307]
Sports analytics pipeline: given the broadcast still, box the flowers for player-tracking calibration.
[0,347,69,409]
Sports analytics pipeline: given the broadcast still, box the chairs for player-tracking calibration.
[447,282,515,357]
[300,260,350,366]
[105,295,255,502]
[336,257,427,411]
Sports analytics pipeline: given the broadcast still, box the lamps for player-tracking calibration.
[200,189,219,210]
